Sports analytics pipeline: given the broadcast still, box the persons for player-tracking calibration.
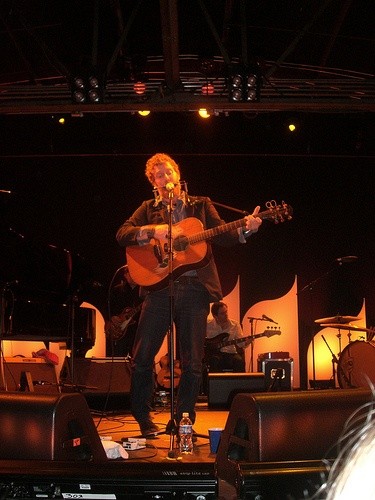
[117,154,262,442]
[206,302,256,373]
[112,267,138,359]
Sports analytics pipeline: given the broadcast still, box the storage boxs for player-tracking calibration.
[258,359,293,391]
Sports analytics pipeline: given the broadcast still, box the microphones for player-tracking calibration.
[165,183,175,192]
[338,256,357,261]
[32,381,44,385]
[262,315,273,322]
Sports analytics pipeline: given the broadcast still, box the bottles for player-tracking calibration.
[179,411,195,453]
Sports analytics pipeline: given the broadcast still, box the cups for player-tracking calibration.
[208,427,225,453]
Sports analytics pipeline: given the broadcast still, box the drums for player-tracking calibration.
[337,340,375,391]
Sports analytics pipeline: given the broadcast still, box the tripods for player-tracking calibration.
[121,190,209,458]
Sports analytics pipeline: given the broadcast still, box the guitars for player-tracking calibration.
[126,200,294,293]
[104,302,144,347]
[156,324,182,389]
[204,326,282,364]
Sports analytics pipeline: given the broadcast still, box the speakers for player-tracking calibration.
[207,372,375,488]
[0,356,134,461]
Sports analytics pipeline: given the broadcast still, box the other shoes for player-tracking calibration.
[138,419,159,435]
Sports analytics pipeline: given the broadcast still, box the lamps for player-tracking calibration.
[71,73,106,103]
[227,73,260,102]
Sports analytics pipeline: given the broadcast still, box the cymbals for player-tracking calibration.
[314,316,362,324]
[320,323,368,331]
